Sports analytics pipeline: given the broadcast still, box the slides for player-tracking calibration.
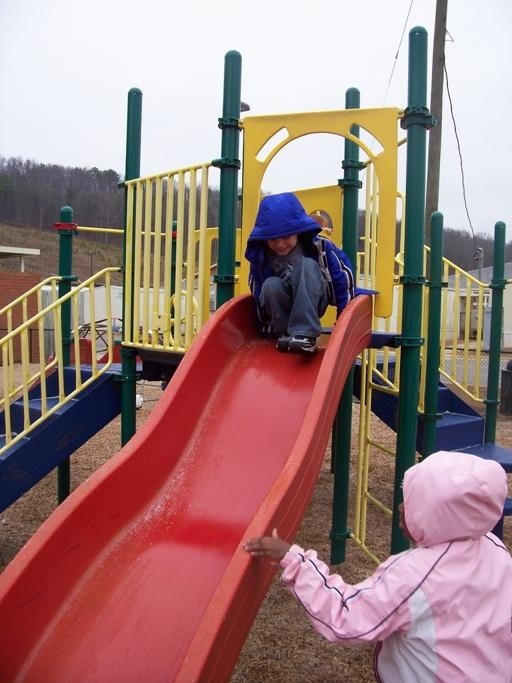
[0,290,371,682]
[27,337,142,380]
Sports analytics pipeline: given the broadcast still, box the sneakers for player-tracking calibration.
[276,332,318,352]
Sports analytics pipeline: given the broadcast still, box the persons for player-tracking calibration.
[242,448,511,681]
[243,192,355,351]
